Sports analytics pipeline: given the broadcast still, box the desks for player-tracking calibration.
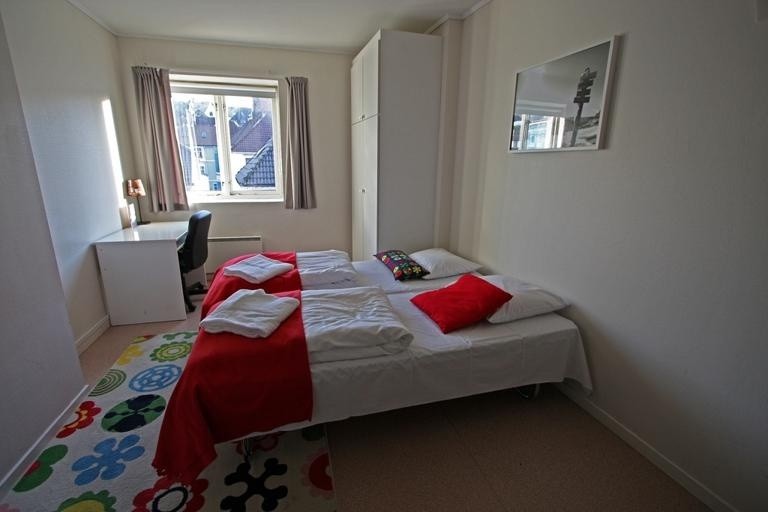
[92,221,207,327]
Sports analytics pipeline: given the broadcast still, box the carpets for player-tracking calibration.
[0,331,338,512]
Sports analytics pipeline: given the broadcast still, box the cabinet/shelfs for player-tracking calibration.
[349,28,443,261]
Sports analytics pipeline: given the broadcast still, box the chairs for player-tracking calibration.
[177,209,212,312]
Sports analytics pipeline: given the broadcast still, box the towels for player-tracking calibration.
[198,287,301,337]
[223,254,294,283]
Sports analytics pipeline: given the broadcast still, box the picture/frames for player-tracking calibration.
[507,35,619,154]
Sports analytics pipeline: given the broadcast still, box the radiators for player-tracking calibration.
[204,236,263,273]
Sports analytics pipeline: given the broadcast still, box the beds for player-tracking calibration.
[151,277,593,488]
[200,249,485,320]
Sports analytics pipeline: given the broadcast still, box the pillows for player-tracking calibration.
[372,249,430,280]
[409,272,514,334]
[443,274,569,323]
[408,247,484,279]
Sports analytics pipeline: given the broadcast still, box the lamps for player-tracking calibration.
[127,179,151,224]
